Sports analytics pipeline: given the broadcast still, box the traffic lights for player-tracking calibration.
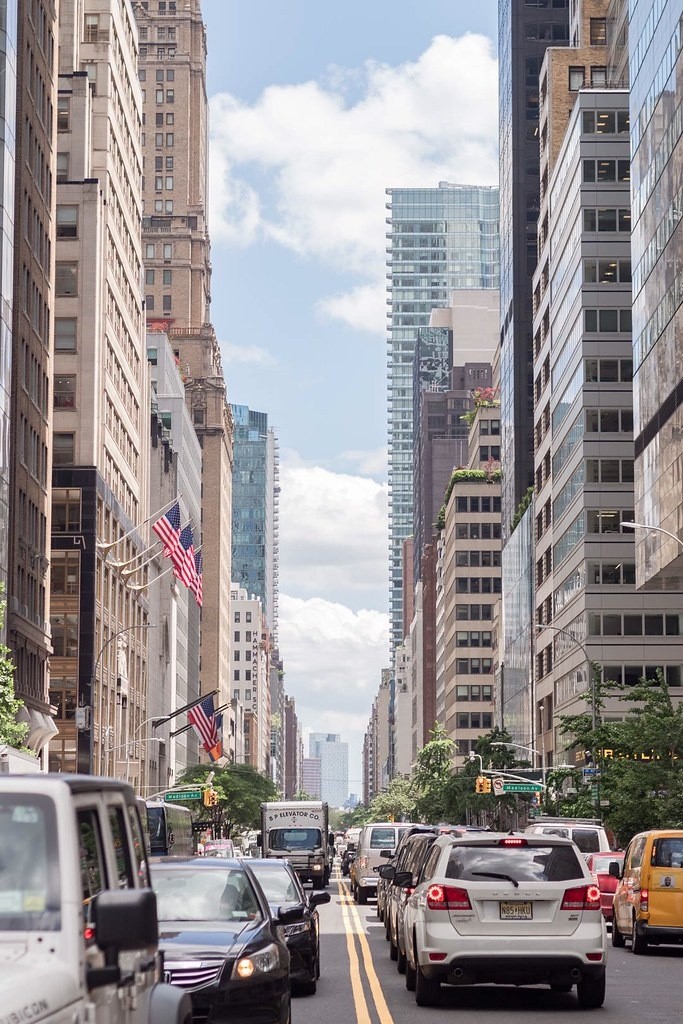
[475,776,491,794]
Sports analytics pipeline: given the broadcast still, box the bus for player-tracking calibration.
[147,802,193,857]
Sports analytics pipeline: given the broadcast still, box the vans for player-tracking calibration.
[204,839,234,858]
[522,823,611,862]
[611,829,683,955]
[350,823,424,905]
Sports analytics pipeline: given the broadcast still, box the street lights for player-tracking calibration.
[490,706,547,804]
[125,716,172,783]
[619,522,683,548]
[91,624,158,774]
[104,726,166,779]
[535,624,596,778]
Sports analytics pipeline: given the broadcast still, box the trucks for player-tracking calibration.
[256,800,335,890]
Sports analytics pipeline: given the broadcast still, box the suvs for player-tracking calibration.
[0,772,194,1023]
[144,858,306,1024]
[372,826,611,1009]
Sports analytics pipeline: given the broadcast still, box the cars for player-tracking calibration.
[234,829,262,858]
[328,825,364,877]
[243,859,331,997]
[584,853,626,925]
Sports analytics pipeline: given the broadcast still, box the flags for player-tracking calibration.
[164,525,196,586]
[203,714,223,752]
[175,551,202,607]
[187,695,220,752]
[152,502,181,557]
